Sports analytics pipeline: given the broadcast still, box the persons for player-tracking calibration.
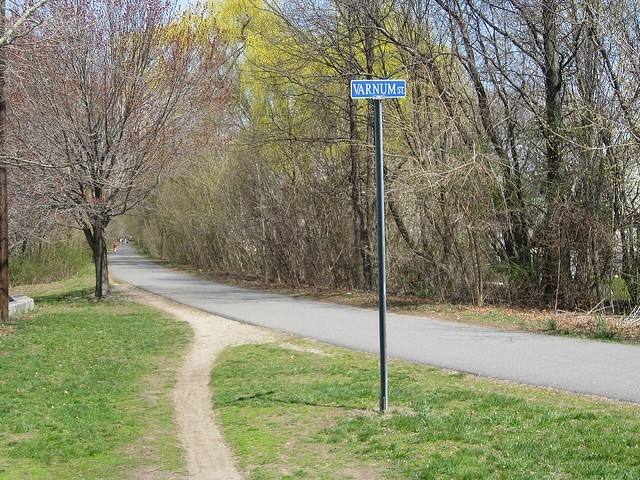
[112,242,117,253]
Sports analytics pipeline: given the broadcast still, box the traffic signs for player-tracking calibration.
[351,81,407,99]
